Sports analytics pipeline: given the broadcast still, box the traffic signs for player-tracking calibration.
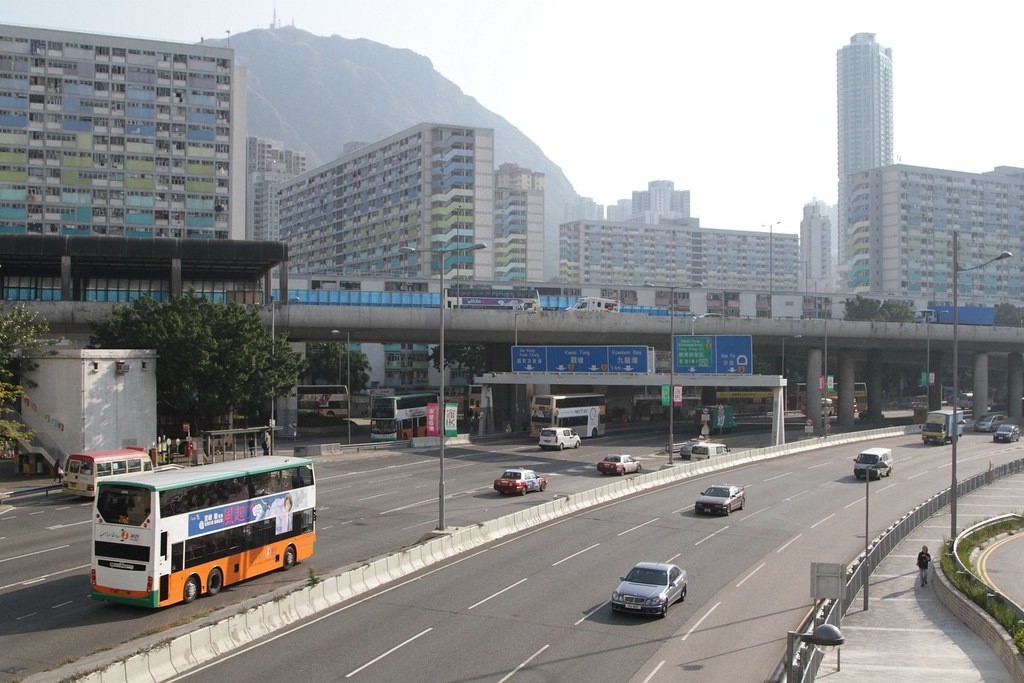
[546,344,609,374]
[607,345,648,374]
[671,334,753,377]
[511,345,547,374]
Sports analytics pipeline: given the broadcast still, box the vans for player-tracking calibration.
[855,447,894,480]
[538,426,582,451]
[975,412,1008,433]
[564,297,620,314]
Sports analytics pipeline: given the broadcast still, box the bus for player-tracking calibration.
[528,392,607,439]
[716,391,774,419]
[631,389,703,422]
[369,391,439,442]
[87,455,320,608]
[297,385,348,419]
[61,445,154,498]
[444,286,543,312]
[794,382,868,411]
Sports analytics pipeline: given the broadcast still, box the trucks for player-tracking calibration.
[696,405,737,434]
[919,409,964,446]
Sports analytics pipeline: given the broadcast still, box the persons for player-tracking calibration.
[262,438,269,456]
[249,437,255,458]
[917,546,931,587]
[53,458,62,484]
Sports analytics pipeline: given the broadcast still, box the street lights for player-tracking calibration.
[863,461,889,610]
[514,311,537,428]
[331,330,351,444]
[782,335,803,378]
[254,296,300,456]
[761,220,781,319]
[399,243,487,530]
[950,230,1013,541]
[926,310,950,413]
[786,624,846,683]
[643,281,703,465]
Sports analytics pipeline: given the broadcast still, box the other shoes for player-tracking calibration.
[925,581,928,584]
[921,584,924,586]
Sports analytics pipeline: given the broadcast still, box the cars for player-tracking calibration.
[801,396,865,430]
[597,453,642,476]
[993,423,1021,443]
[700,312,726,318]
[694,483,745,517]
[913,386,994,410]
[612,561,688,619]
[494,467,548,496]
